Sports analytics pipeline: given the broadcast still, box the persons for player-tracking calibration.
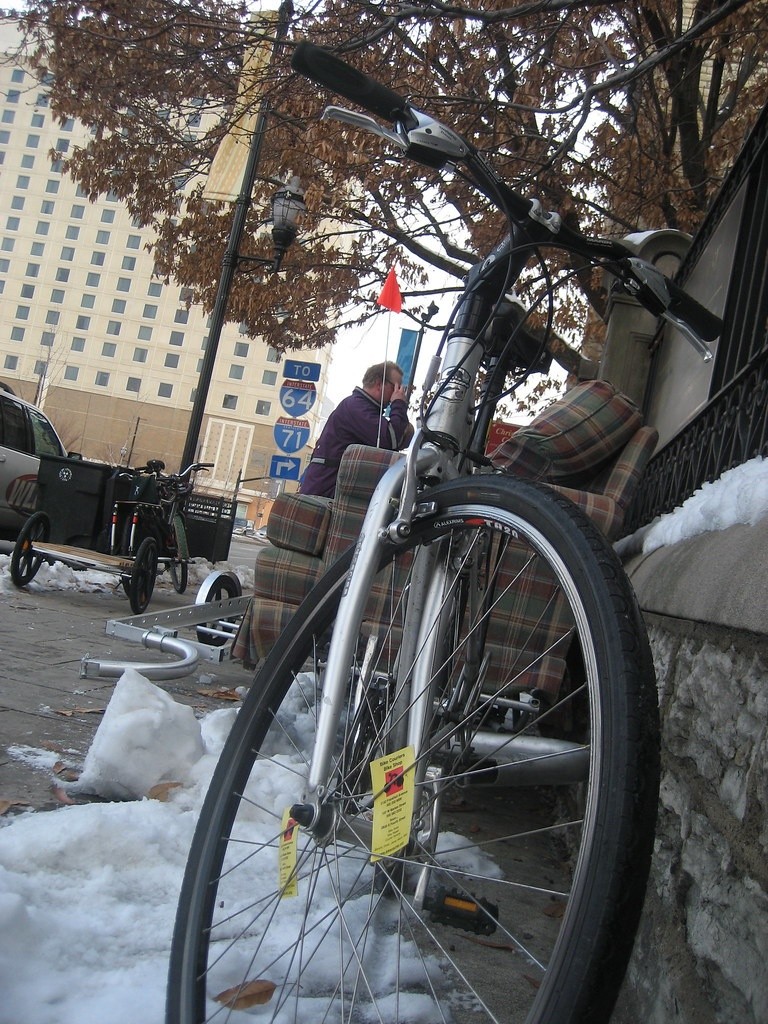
[298,361,415,496]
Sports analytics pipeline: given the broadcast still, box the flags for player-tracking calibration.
[376,266,403,315]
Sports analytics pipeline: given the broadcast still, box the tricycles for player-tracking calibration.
[10,458,216,616]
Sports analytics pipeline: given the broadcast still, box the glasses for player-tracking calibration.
[386,378,402,392]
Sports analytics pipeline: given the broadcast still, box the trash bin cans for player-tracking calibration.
[32,451,171,557]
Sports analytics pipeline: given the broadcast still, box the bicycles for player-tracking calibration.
[161,39,724,1023]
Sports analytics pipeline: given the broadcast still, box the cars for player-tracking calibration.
[255,525,268,539]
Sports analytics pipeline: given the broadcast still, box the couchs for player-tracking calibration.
[232,378,660,731]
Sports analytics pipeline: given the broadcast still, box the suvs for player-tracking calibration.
[0,380,82,541]
